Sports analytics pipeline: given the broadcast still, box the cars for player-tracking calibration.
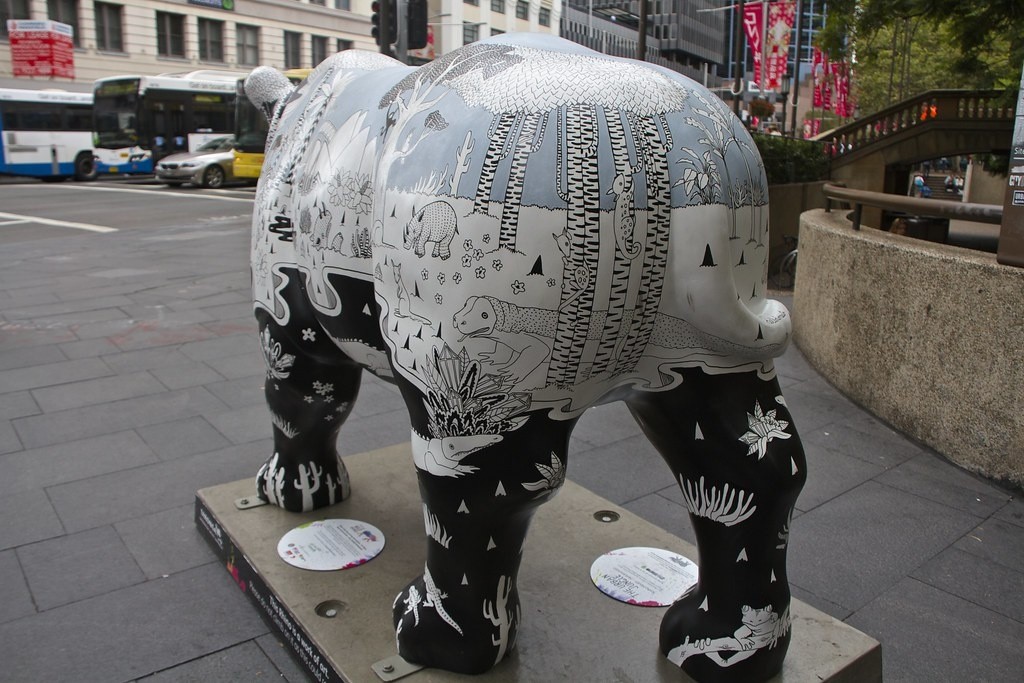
[154,135,238,189]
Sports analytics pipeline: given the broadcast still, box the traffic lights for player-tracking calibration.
[370,0,397,46]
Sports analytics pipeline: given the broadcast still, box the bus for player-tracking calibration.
[92,70,238,175]
[232,69,311,182]
[0,89,136,181]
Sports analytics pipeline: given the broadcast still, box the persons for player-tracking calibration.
[825,101,968,199]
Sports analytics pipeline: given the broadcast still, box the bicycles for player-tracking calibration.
[771,233,799,292]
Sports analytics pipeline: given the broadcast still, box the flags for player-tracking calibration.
[735,0,859,139]
